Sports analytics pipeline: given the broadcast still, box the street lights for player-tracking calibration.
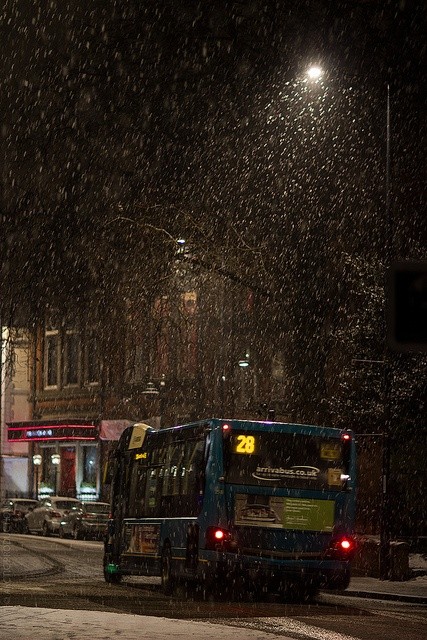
[304,62,401,583]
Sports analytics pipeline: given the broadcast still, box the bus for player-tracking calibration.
[104,416,358,603]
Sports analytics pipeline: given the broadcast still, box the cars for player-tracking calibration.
[59,502,111,540]
[21,496,83,535]
[0,498,38,533]
